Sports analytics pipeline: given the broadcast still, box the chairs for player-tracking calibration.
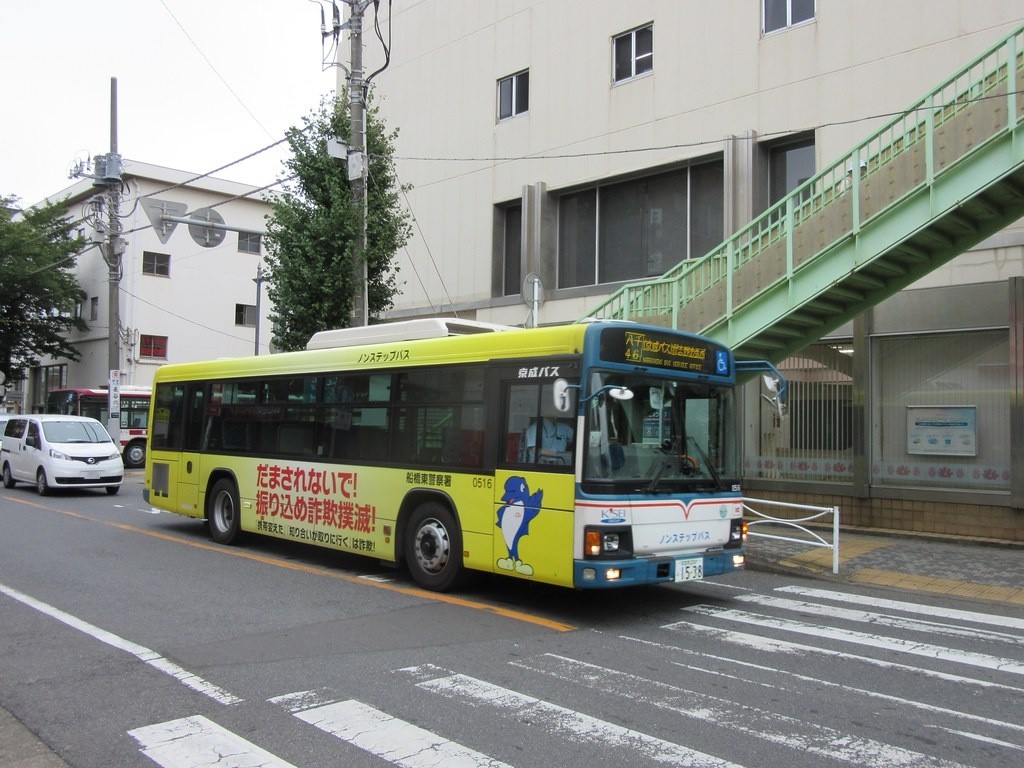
[442,426,467,463]
[191,421,405,464]
[133,418,141,428]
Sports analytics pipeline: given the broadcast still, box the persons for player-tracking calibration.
[521,396,575,466]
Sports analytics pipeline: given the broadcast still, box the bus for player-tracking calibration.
[143,316,749,593]
[47,385,369,469]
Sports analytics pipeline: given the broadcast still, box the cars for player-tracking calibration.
[0,414,125,496]
